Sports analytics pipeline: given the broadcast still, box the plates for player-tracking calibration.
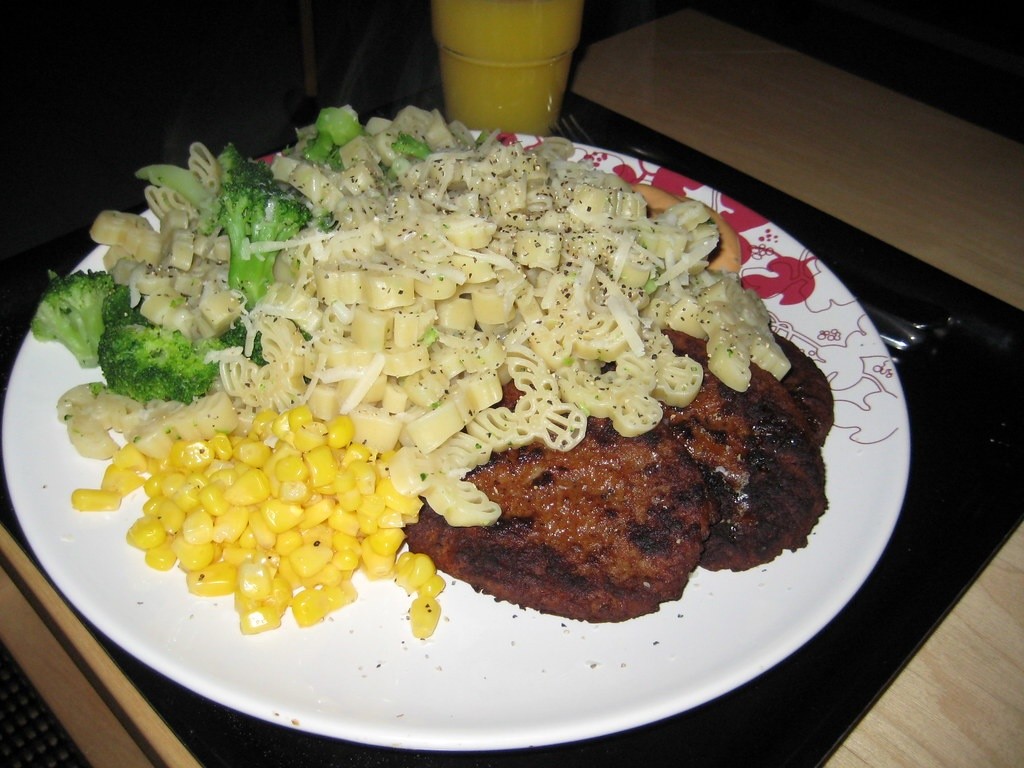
[2,127,911,751]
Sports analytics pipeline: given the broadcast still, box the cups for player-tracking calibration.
[431,0,583,137]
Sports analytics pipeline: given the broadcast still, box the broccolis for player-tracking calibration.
[30,106,433,406]
